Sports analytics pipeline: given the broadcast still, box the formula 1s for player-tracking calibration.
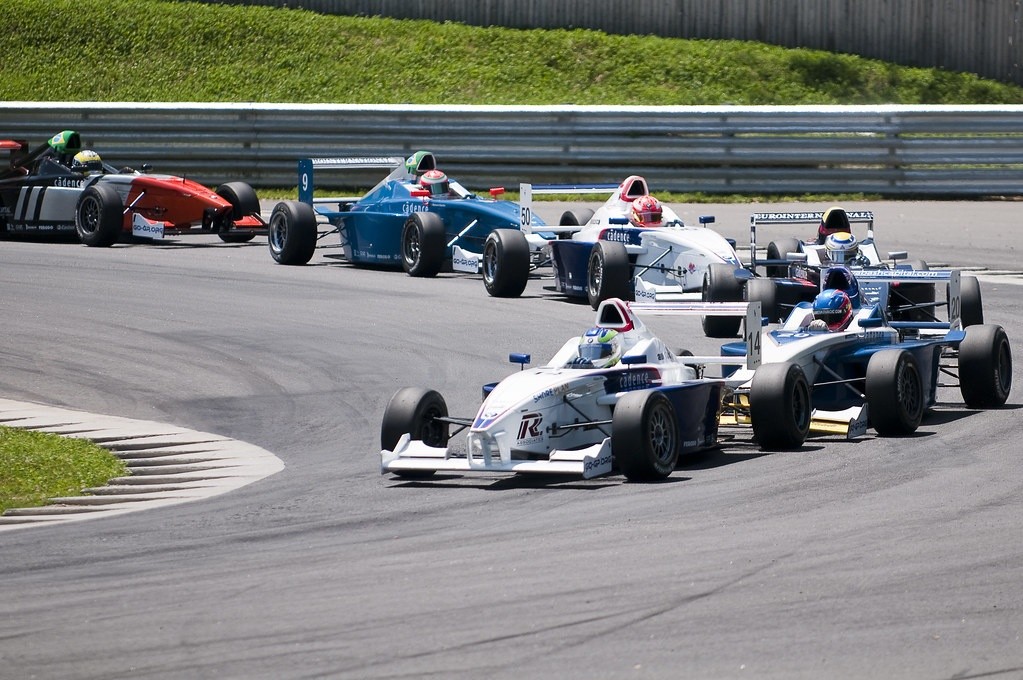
[481,175,800,311]
[665,263,1012,438]
[703,206,983,351]
[381,295,811,485]
[0,127,270,248]
[269,149,597,278]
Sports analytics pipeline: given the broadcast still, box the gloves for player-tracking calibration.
[462,193,476,199]
[807,319,826,332]
[566,358,594,370]
[853,256,870,268]
[670,220,684,228]
[120,167,133,174]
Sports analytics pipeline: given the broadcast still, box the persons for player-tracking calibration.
[808,289,854,332]
[629,196,663,227]
[420,169,453,201]
[71,150,104,175]
[822,232,870,267]
[565,328,627,369]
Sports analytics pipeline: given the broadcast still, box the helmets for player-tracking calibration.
[630,196,663,227]
[812,289,852,332]
[419,170,450,198]
[578,327,622,368]
[72,149,102,177]
[825,232,858,262]
